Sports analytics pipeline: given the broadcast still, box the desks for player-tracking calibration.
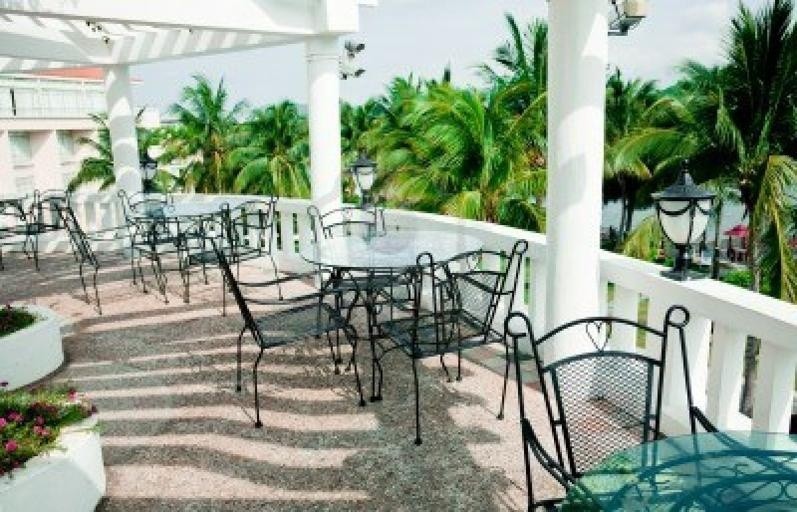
[553,429,797,512]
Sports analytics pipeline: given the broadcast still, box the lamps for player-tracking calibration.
[139,151,158,196]
[646,158,717,283]
[347,147,380,211]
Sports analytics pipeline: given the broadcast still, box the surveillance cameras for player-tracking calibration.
[609,0,649,29]
[341,62,364,79]
[345,38,366,55]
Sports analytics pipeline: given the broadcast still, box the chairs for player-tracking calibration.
[499,305,797,512]
